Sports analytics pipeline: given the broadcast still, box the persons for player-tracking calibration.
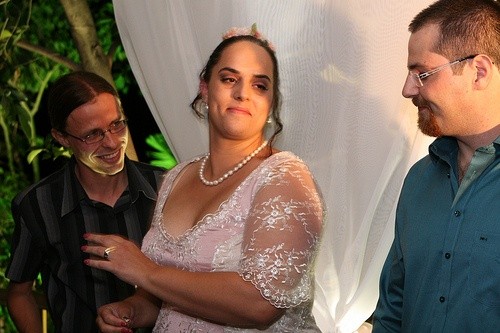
[370,0,500,333]
[81,22,326,333]
[8,72,169,333]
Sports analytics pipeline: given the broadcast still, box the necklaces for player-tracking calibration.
[199,140,267,186]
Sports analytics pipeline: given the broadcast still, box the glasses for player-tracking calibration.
[59,115,128,144]
[410,53,494,87]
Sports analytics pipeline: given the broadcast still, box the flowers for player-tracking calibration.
[222,22,277,54]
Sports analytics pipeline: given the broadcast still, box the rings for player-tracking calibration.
[104,246,117,259]
